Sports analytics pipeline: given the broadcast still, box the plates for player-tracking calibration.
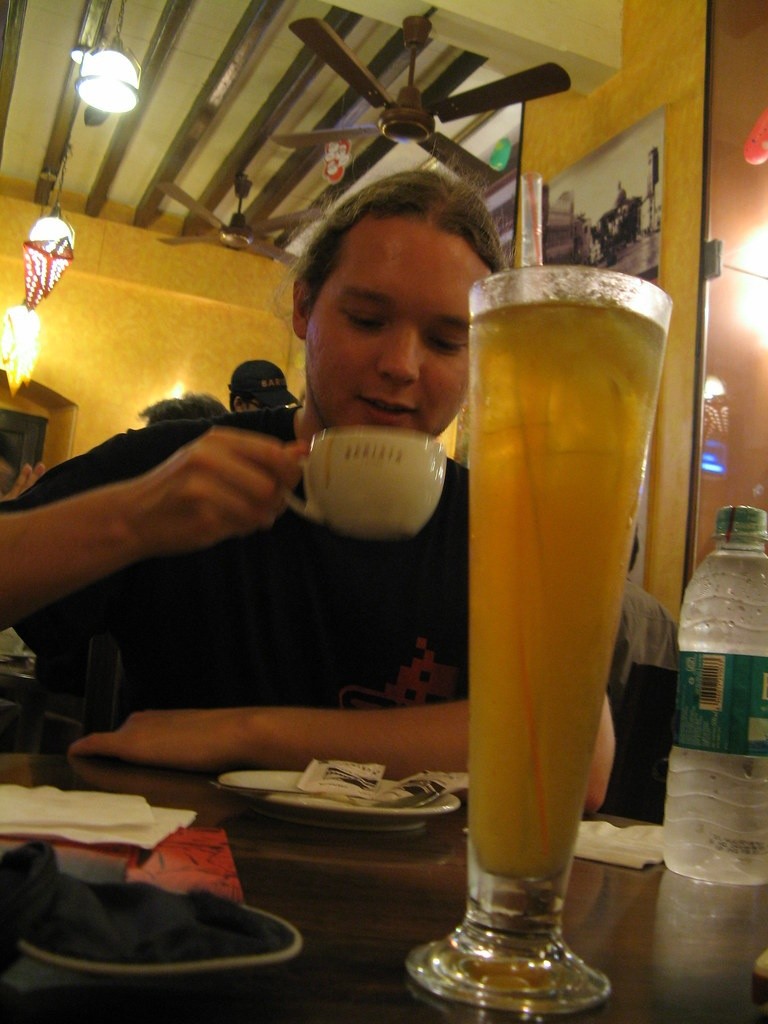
[222,769,461,830]
[19,905,303,976]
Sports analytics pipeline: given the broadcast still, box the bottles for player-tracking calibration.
[649,505,768,1024]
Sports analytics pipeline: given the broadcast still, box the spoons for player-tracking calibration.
[208,778,444,808]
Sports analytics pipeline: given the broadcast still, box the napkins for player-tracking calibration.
[0,781,194,851]
[571,821,670,869]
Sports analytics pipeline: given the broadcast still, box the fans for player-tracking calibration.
[156,175,324,266]
[271,4,572,186]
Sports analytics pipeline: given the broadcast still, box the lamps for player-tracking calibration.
[72,1,142,113]
[2,153,74,388]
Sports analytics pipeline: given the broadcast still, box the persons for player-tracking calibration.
[1,461,44,503]
[227,359,301,412]
[0,175,614,816]
[606,529,676,718]
[139,394,229,424]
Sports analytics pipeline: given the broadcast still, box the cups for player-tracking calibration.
[279,425,447,542]
[401,262,671,1012]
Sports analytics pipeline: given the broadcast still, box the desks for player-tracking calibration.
[0,751,768,1024]
